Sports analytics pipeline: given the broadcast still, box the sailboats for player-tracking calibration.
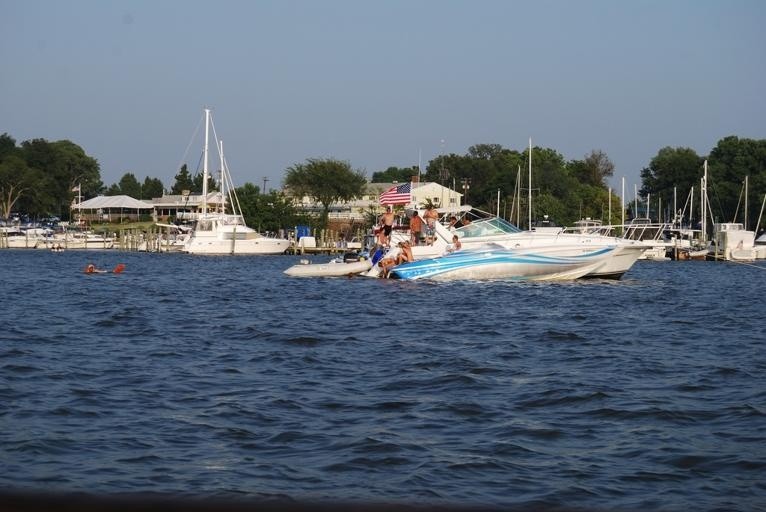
[328,137,766,283]
[138,109,290,254]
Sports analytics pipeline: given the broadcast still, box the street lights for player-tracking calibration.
[460,177,471,205]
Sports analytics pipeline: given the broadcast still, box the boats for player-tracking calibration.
[0,213,115,249]
[282,251,374,279]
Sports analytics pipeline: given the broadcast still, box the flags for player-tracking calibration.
[70,185,79,192]
[379,183,412,206]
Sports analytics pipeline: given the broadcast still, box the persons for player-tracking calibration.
[461,217,472,226]
[445,216,462,229]
[85,263,125,274]
[447,235,461,253]
[369,205,439,280]
